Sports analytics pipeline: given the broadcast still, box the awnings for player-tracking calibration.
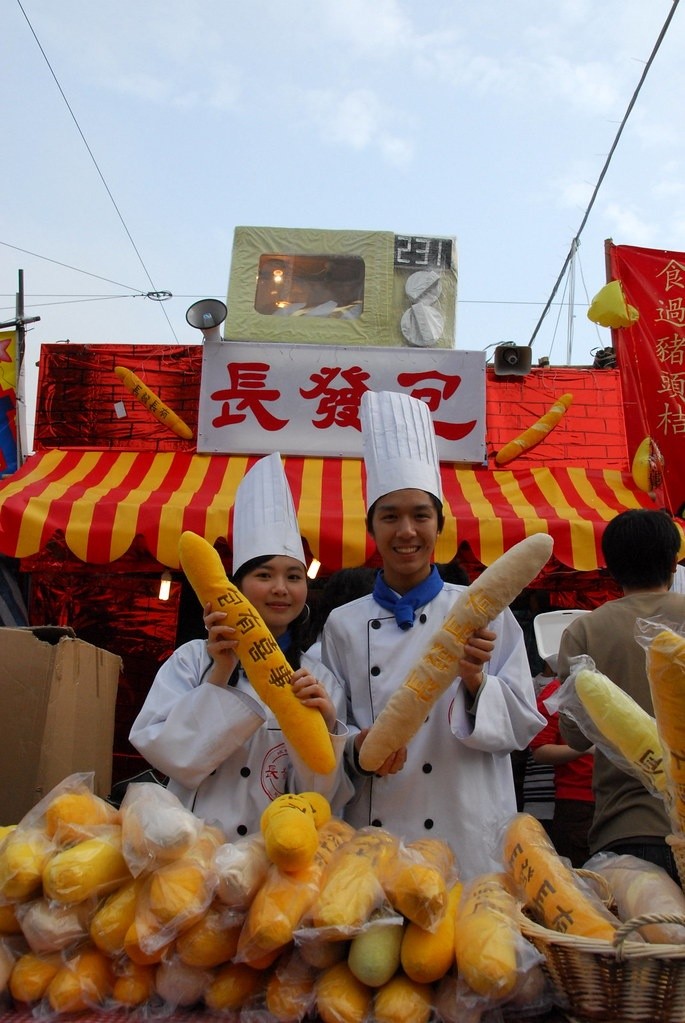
[0,447,685,574]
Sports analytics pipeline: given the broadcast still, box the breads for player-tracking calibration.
[496,394,572,464]
[114,366,193,439]
[0,531,685,1023]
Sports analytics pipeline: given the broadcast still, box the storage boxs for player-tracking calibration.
[534,610,593,674]
[0,624,125,827]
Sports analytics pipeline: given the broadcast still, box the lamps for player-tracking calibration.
[269,259,289,283]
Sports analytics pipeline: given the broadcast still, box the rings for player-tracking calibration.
[205,625,209,631]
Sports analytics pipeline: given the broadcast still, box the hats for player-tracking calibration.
[233,451,307,576]
[360,390,443,514]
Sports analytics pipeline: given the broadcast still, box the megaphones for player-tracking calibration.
[494,345,532,376]
[185,298,227,341]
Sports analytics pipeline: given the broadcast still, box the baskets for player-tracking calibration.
[515,868,685,1023]
[665,834,685,894]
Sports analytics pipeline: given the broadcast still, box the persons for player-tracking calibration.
[128,515,348,843]
[299,454,548,887]
[529,508,685,888]
